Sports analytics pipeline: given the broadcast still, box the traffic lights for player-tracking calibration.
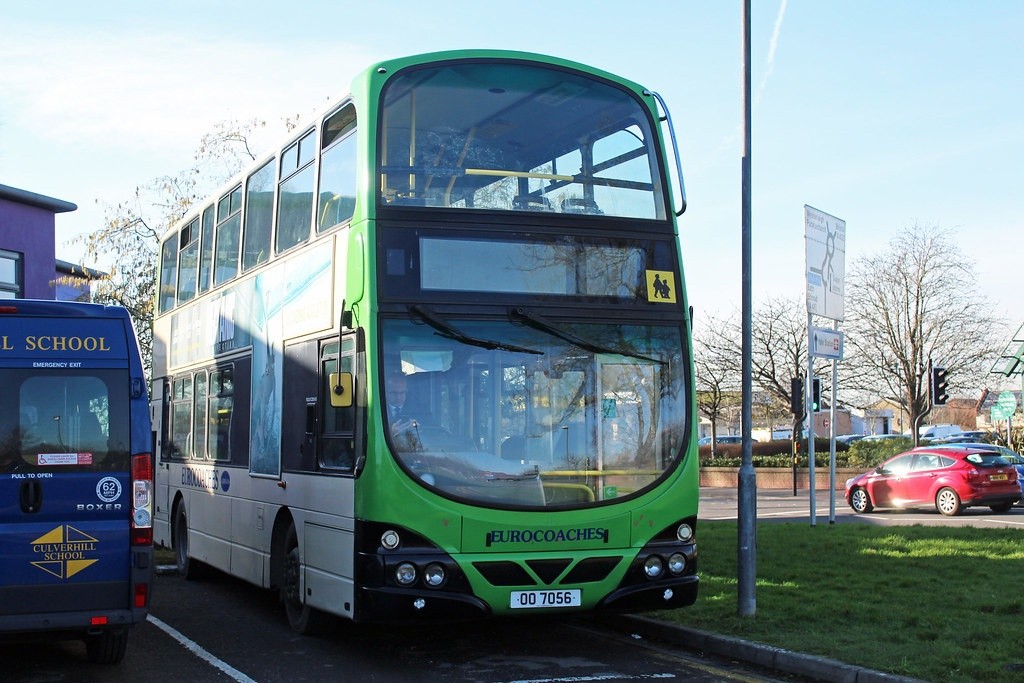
[793,441,800,455]
[805,377,822,414]
[932,366,950,407]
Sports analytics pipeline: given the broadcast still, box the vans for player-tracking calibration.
[0,295,155,666]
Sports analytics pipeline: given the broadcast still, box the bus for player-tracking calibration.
[148,47,702,636]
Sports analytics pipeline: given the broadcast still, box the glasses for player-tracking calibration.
[387,390,406,395]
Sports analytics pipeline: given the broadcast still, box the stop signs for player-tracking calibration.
[824,418,829,428]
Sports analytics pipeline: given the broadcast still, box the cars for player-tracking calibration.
[915,442,1024,500]
[697,435,758,446]
[843,444,1022,517]
[835,422,998,444]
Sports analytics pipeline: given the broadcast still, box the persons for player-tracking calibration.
[385,374,437,453]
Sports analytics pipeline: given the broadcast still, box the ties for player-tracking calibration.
[394,407,400,418]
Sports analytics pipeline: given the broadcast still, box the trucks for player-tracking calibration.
[772,424,819,440]
